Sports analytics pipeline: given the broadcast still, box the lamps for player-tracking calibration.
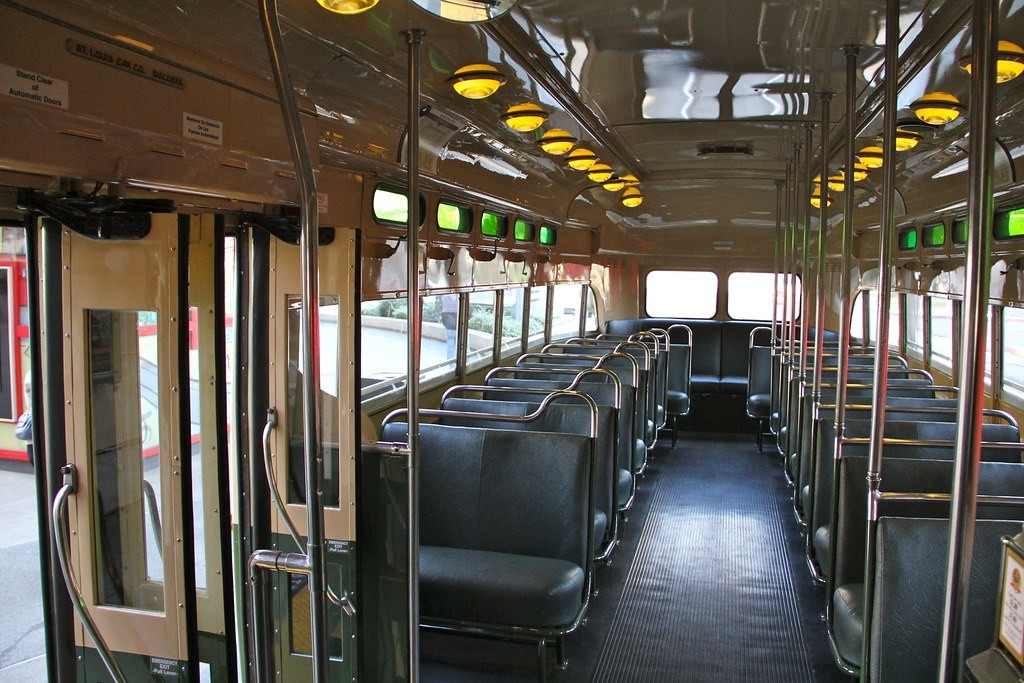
[319,0,1024,212]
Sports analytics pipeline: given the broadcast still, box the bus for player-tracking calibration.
[0,244,237,476]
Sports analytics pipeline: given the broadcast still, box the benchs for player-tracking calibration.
[382,319,1024,683]
